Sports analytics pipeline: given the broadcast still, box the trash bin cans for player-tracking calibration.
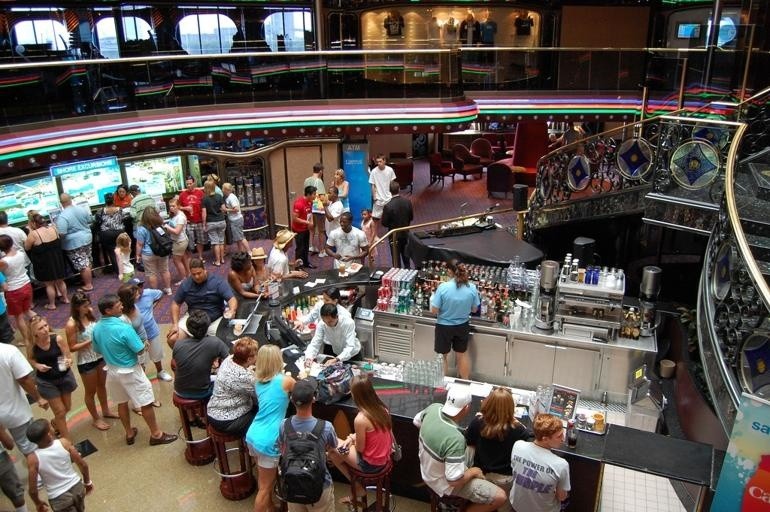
[512,185,528,211]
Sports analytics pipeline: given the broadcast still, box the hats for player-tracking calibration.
[273,230,298,250]
[441,386,473,417]
[128,277,144,286]
[249,246,268,260]
[290,376,318,407]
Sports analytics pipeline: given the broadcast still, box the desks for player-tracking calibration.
[491,146,514,160]
[371,160,414,195]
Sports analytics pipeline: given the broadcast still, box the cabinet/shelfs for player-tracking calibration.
[373,265,660,406]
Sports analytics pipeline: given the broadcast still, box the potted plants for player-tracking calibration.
[497,134,507,148]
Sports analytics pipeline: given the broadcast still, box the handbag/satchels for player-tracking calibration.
[383,408,403,462]
[318,363,354,406]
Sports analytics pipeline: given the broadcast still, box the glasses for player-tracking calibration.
[29,315,42,324]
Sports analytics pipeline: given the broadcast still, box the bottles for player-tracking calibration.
[619,308,641,340]
[562,253,624,319]
[568,420,577,448]
[237,184,262,207]
[282,295,316,322]
[416,260,513,326]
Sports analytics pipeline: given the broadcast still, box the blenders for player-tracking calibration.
[534,261,559,330]
[637,266,662,337]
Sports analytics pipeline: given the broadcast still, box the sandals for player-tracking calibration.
[50,419,60,438]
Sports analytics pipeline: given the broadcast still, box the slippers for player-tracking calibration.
[133,409,143,416]
[92,422,112,430]
[152,400,161,408]
[43,303,57,311]
[75,287,94,292]
[339,496,366,508]
[212,262,221,266]
[103,412,120,419]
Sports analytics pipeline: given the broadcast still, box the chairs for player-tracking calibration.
[430,152,455,185]
[389,151,409,190]
[170,358,205,425]
[486,162,514,200]
[450,144,484,182]
[470,138,496,173]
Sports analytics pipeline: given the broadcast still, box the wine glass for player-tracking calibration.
[379,360,405,381]
[189,206,193,216]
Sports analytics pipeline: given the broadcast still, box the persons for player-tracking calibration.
[384,10,534,82]
[0,154,572,512]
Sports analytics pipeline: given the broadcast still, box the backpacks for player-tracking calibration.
[143,224,172,257]
[274,415,328,505]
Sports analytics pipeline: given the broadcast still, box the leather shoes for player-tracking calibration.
[126,428,137,445]
[308,264,317,268]
[150,432,178,446]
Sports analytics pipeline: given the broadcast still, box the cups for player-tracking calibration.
[57,357,68,372]
[339,264,345,274]
[405,354,443,396]
[577,414,605,432]
[536,385,552,410]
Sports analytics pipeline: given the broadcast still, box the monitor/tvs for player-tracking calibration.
[274,315,307,350]
[678,23,701,38]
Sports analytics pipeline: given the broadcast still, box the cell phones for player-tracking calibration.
[338,446,350,453]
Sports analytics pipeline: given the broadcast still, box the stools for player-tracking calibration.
[423,484,468,512]
[344,460,393,512]
[267,456,288,512]
[208,424,258,500]
[172,392,216,466]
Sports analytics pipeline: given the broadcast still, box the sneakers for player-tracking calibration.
[156,370,172,381]
[37,480,44,490]
[161,287,172,296]
[318,252,329,257]
[174,281,181,286]
[309,246,320,254]
